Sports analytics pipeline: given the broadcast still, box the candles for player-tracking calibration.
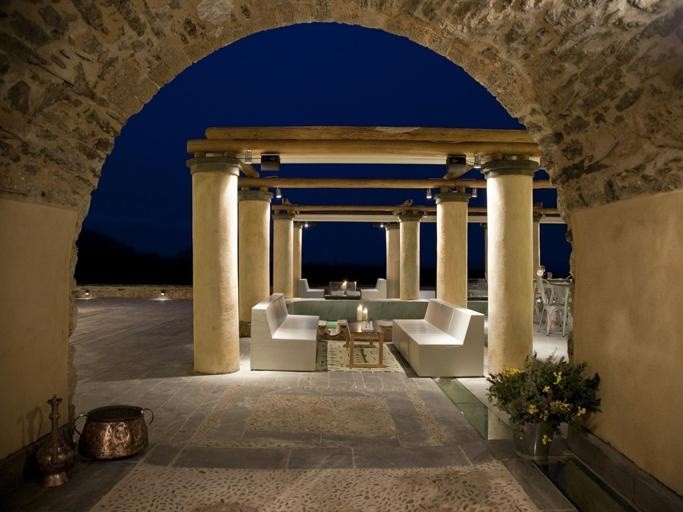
[356,304,363,320]
[341,281,346,289]
[363,307,368,320]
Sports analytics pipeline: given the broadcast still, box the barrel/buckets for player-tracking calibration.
[511,417,558,462]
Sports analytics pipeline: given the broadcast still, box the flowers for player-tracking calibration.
[485,348,603,445]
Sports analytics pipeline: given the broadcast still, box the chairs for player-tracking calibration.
[533,275,573,336]
[298,278,325,298]
[361,278,387,299]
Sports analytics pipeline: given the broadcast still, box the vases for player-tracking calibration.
[512,421,554,461]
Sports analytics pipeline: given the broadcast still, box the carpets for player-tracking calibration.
[317,340,408,374]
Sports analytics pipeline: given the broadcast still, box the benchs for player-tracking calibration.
[392,299,485,377]
[250,293,320,372]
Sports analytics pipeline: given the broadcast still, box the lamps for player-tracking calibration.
[276,189,282,199]
[160,289,165,296]
[471,189,478,197]
[535,265,545,276]
[426,188,433,198]
[84,290,90,295]
